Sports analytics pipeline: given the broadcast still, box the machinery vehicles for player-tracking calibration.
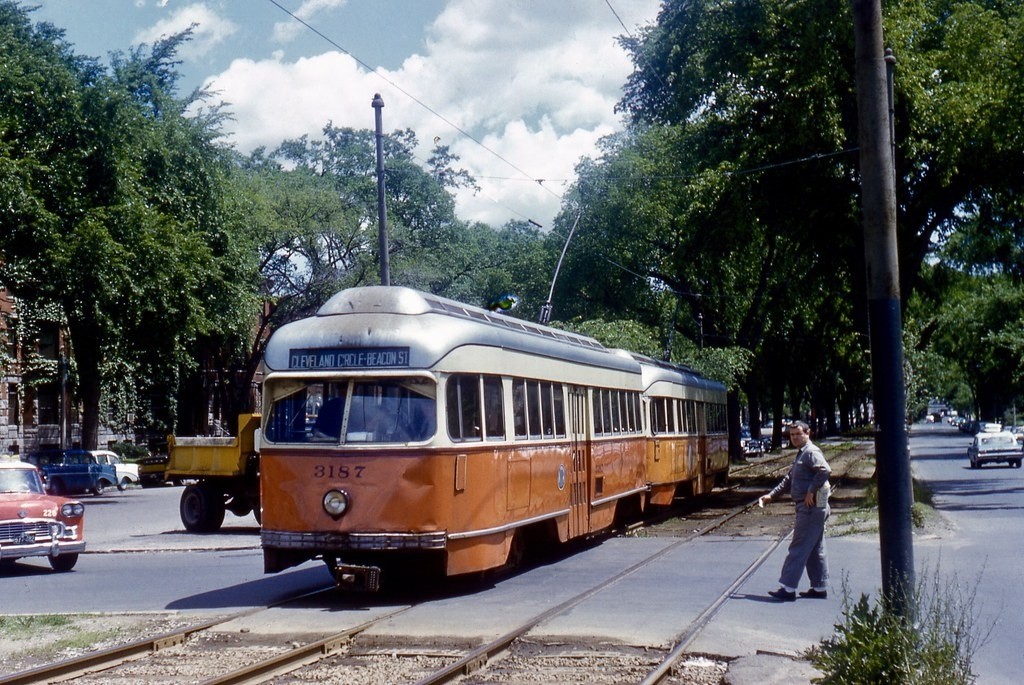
[161,412,262,535]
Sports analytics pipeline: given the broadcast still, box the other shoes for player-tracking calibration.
[799,589,827,598]
[768,588,796,601]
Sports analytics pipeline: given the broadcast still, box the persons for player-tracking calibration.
[310,381,367,441]
[758,423,832,601]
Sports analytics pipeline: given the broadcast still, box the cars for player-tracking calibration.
[26,450,118,496]
[0,462,85,570]
[967,432,1024,470]
[925,413,1024,442]
[736,413,854,459]
[87,451,139,491]
[141,456,186,487]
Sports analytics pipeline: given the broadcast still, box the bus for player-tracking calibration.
[259,284,728,595]
